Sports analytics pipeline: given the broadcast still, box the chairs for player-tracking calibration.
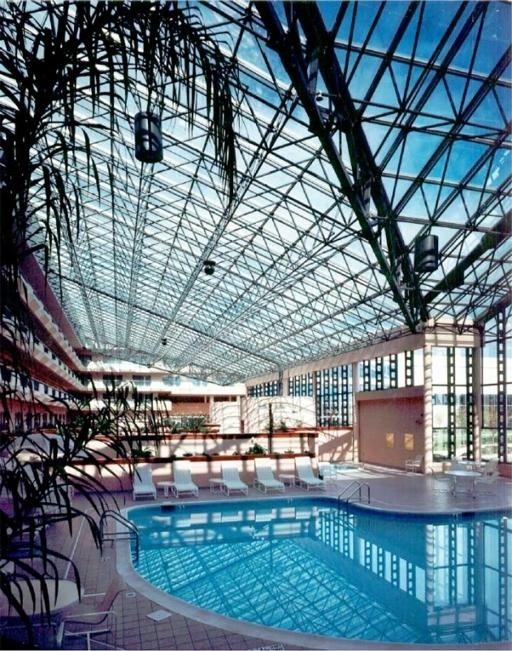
[133,504,315,530]
[56,574,128,651]
[0,460,75,560]
[132,456,338,502]
[405,454,499,498]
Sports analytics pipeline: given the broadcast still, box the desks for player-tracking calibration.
[0,578,84,648]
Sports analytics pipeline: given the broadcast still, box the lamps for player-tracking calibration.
[134,109,164,163]
[414,234,438,273]
[203,260,215,276]
[161,338,167,345]
